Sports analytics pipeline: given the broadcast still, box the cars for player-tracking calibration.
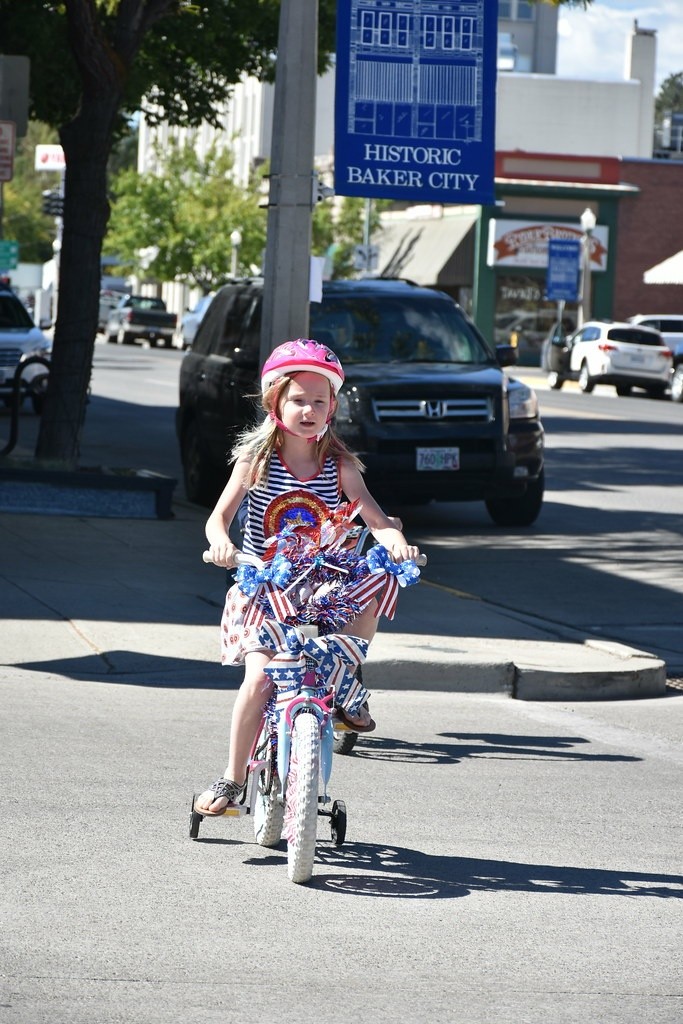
[0,277,52,412]
[491,309,575,349]
[176,292,217,351]
[628,314,683,365]
[540,318,672,400]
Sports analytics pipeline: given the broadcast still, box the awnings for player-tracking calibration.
[643,249,683,284]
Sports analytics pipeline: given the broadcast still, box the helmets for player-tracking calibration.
[261,340,344,394]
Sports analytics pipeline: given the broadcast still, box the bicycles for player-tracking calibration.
[186,549,427,884]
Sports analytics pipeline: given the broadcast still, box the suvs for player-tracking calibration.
[173,278,548,530]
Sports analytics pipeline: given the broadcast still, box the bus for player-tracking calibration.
[100,260,133,332]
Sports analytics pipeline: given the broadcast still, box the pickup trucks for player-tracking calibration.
[105,297,176,348]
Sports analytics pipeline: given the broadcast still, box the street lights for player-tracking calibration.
[231,231,242,278]
[581,207,596,326]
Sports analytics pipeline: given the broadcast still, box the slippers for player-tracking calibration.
[337,703,377,733]
[194,774,249,817]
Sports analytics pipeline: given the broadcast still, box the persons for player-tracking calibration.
[510,326,522,365]
[194,339,419,817]
